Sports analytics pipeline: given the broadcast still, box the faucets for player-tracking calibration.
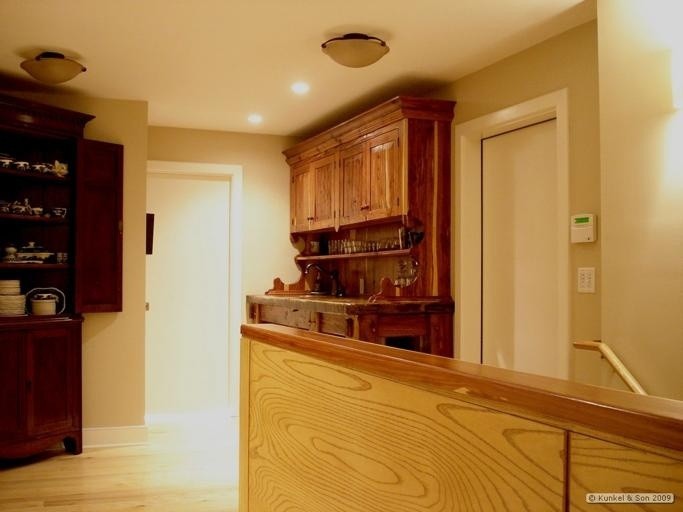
[304,263,334,275]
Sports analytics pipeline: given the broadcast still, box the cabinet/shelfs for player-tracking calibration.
[247,95,456,359]
[0,92,123,472]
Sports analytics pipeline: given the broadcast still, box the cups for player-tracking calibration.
[56,252,68,264]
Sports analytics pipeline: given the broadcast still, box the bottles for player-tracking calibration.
[328,238,401,254]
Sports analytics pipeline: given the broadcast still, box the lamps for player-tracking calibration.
[20,50,88,84]
[322,33,391,69]
